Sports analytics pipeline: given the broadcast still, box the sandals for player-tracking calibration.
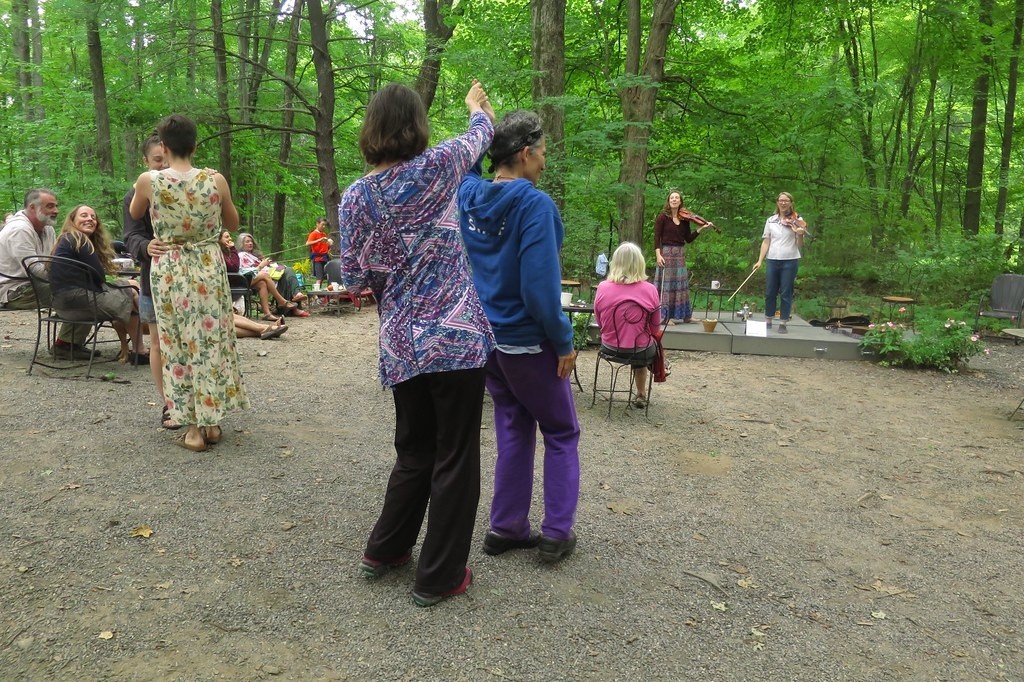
[161,404,182,428]
[261,324,288,340]
[275,314,285,338]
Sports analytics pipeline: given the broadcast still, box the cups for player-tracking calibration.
[314,284,320,290]
[333,284,339,291]
[331,282,337,288]
[710,281,720,289]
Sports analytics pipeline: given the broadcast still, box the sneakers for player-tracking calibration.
[483,527,542,556]
[538,529,577,562]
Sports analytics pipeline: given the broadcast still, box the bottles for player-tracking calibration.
[742,304,749,321]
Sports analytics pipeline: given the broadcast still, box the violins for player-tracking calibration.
[678,207,721,234]
[780,210,817,243]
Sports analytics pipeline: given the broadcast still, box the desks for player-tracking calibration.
[561,302,594,393]
[307,289,357,318]
[998,328,1024,420]
[561,279,581,300]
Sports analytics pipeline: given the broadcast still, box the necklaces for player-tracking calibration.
[673,214,677,219]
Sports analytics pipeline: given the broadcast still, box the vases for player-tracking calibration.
[561,292,573,306]
[702,320,717,332]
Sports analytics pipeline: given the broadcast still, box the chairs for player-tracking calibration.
[815,274,849,321]
[876,261,924,336]
[587,299,672,423]
[974,274,1024,345]
[585,252,600,304]
[0,240,376,381]
[690,252,738,321]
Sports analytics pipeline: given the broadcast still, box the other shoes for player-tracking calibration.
[358,547,412,580]
[684,318,701,323]
[291,310,310,317]
[662,320,675,326]
[664,359,672,375]
[278,301,295,309]
[412,566,473,606]
[635,395,647,407]
[767,322,772,329]
[131,348,151,365]
[51,342,101,359]
[778,324,786,333]
[292,294,308,301]
[263,314,279,321]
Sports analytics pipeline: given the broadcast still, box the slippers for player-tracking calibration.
[199,425,223,444]
[175,430,206,451]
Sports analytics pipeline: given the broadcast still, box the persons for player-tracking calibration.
[594,242,672,409]
[0,189,57,310]
[218,229,309,321]
[0,212,15,232]
[306,217,334,288]
[338,82,494,608]
[753,191,804,334]
[128,280,289,340]
[457,79,581,563]
[123,131,217,429]
[129,115,250,451]
[654,191,713,326]
[49,204,151,366]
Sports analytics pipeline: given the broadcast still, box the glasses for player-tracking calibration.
[778,200,790,203]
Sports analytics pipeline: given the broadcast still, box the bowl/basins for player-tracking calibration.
[701,320,717,332]
[561,292,573,307]
